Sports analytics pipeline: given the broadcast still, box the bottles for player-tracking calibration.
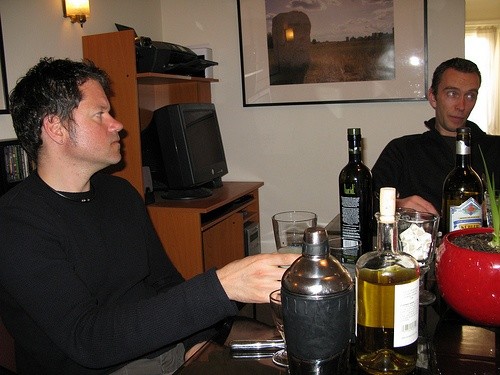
[281,227,354,375]
[442,128,486,237]
[357,213,420,375]
[339,128,373,264]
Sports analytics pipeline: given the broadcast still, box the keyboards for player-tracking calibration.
[199,194,255,224]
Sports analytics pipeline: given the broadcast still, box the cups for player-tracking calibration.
[484,190,500,230]
[272,211,317,269]
[328,238,362,280]
[375,207,420,253]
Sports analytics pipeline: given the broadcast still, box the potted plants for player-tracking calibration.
[431,143,500,326]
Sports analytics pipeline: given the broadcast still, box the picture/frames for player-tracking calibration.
[236,0,431,108]
[0,14,10,114]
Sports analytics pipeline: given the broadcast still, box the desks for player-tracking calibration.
[170,213,500,375]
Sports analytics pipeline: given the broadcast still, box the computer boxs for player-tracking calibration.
[245,222,261,258]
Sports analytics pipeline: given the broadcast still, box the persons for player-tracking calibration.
[370,58,500,238]
[0,58,303,375]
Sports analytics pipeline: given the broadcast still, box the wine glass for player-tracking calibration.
[269,290,291,367]
[397,212,440,305]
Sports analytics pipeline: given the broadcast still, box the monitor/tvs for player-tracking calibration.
[140,102,228,201]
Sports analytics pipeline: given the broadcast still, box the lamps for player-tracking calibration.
[62,0,90,30]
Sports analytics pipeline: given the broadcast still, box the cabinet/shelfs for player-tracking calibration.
[82,31,267,284]
[0,139,32,197]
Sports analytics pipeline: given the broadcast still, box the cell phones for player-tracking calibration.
[229,340,284,350]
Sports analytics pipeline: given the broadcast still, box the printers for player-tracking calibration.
[114,23,219,78]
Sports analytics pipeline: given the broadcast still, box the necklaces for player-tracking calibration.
[49,187,90,203]
